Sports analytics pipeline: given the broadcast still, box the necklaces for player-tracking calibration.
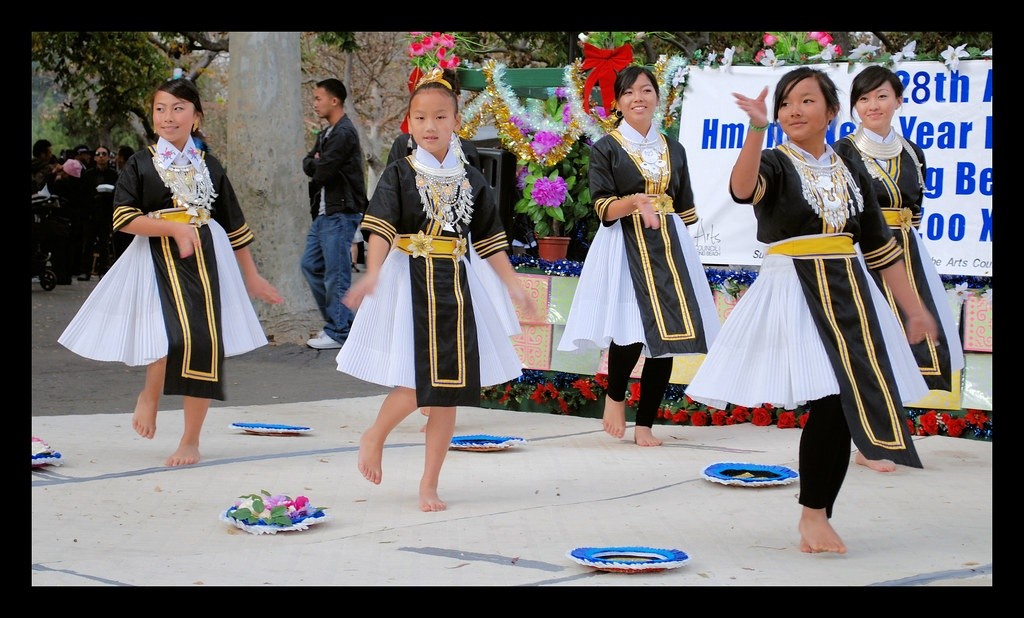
[152,153,219,227]
[411,159,474,233]
[785,146,864,228]
[616,130,670,182]
[854,125,927,190]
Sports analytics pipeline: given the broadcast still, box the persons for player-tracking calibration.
[683,66,937,553]
[57,78,282,465]
[834,66,966,472]
[557,66,721,446]
[343,69,536,512]
[31,139,137,285]
[303,78,368,349]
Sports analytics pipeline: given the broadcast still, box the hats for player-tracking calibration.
[61,159,82,178]
[73,144,90,157]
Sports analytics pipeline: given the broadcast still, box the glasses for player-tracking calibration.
[95,151,108,156]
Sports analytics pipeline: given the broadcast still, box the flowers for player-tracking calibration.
[662,51,717,113]
[939,42,971,71]
[755,32,842,64]
[232,489,324,527]
[848,42,882,60]
[476,373,992,444]
[512,88,591,235]
[722,47,736,70]
[406,32,513,77]
[889,39,919,64]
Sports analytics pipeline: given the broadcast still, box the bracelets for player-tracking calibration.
[749,120,770,131]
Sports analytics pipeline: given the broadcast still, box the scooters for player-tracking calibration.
[32,195,59,290]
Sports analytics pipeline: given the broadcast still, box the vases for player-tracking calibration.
[533,234,571,262]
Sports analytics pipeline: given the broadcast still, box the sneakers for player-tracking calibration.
[306,331,343,349]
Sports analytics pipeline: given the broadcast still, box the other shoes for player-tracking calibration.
[55,277,72,285]
[77,273,90,281]
